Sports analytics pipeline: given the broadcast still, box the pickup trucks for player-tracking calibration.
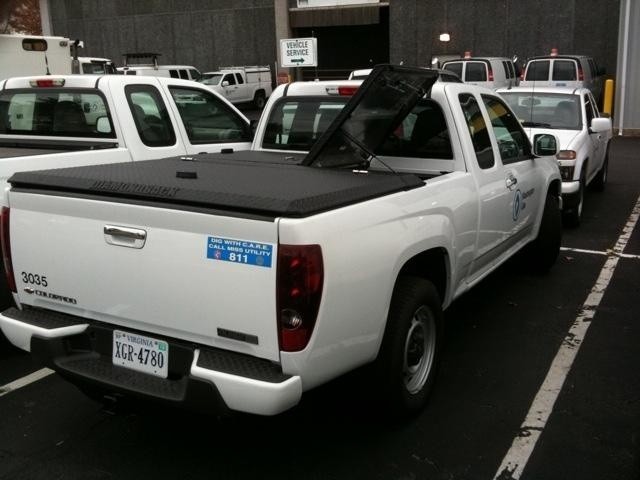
[493,85,613,224]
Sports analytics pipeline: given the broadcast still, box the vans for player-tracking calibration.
[0,35,72,79]
[518,54,602,100]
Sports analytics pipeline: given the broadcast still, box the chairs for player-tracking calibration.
[53,101,87,131]
[317,109,341,135]
[416,112,449,143]
[554,101,577,123]
